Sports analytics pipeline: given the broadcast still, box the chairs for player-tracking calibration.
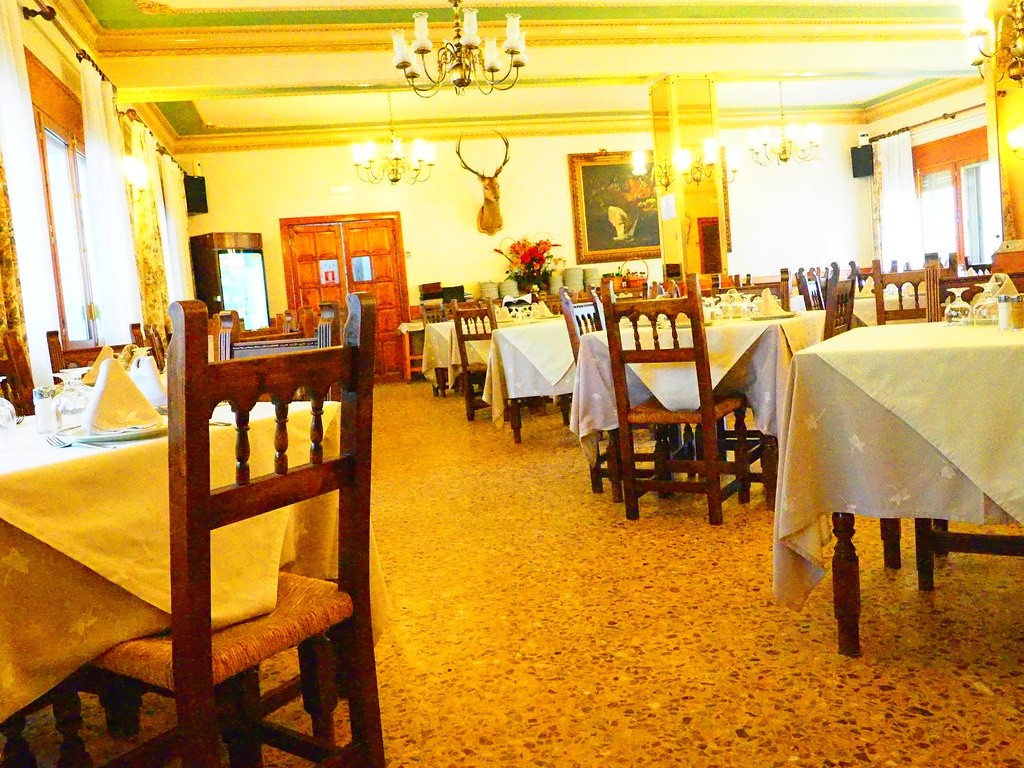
[0,292,386,768]
[419,252,1024,592]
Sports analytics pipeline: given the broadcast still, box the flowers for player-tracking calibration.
[491,237,563,295]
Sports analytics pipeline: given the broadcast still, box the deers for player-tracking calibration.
[457,130,510,236]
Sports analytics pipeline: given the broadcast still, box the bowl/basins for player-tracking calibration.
[480,266,601,301]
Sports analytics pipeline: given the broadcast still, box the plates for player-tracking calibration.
[749,312,796,319]
[670,321,714,328]
[626,321,661,327]
[57,415,168,441]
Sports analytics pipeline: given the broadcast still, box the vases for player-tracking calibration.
[529,283,539,297]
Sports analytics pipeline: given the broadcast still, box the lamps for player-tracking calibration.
[390,0,526,96]
[628,78,719,191]
[352,97,436,186]
[962,0,1024,89]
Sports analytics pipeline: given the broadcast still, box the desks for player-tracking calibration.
[0,398,387,768]
[851,296,927,327]
[422,315,545,398]
[483,315,652,444]
[569,311,829,510]
[773,321,1024,655]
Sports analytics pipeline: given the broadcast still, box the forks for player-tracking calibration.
[15,414,30,424]
[46,435,117,450]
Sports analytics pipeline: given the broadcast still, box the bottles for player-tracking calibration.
[998,293,1024,330]
[603,266,645,280]
[728,274,752,288]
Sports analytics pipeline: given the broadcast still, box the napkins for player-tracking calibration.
[496,306,513,322]
[537,301,556,318]
[757,287,793,318]
[975,272,1018,317]
[531,301,543,318]
[82,346,113,385]
[861,276,875,297]
[80,359,165,435]
[127,356,168,408]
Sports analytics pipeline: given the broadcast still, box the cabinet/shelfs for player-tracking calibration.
[398,322,425,382]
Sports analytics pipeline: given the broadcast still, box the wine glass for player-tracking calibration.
[702,293,757,320]
[944,282,1002,326]
[0,347,153,429]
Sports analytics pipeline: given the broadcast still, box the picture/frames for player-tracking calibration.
[567,150,662,264]
